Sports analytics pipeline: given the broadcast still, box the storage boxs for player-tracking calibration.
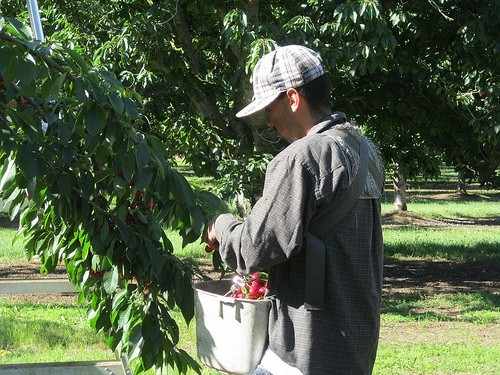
[192,280,272,375]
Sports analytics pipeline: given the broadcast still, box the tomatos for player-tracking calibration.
[205,245,214,253]
[0,77,30,105]
[84,125,90,134]
[229,272,269,300]
[89,182,152,297]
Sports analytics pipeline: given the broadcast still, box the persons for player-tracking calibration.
[202,44,385,375]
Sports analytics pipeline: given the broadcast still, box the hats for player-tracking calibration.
[235,44,325,118]
